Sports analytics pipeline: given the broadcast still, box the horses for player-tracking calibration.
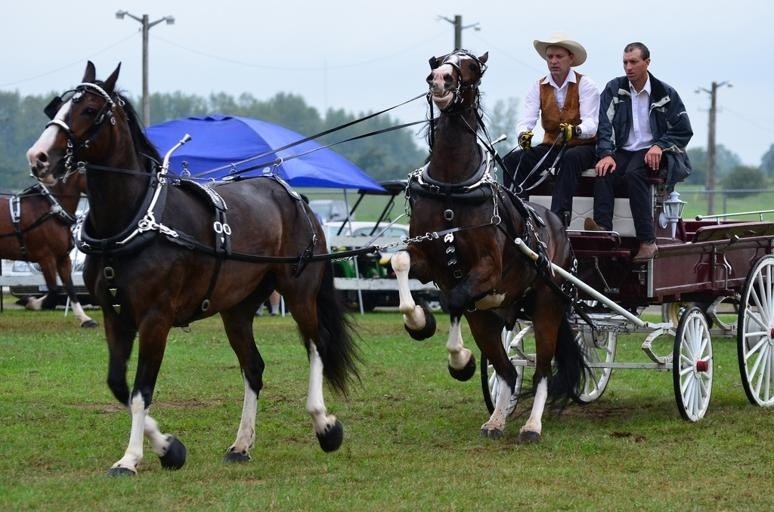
[25,60,373,478]
[0,159,101,329]
[389,49,600,445]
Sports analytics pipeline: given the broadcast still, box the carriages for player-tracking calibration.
[26,50,774,477]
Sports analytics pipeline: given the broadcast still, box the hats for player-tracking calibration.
[533,32,587,66]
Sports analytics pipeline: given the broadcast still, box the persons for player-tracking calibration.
[253,286,282,318]
[500,32,602,230]
[583,42,695,266]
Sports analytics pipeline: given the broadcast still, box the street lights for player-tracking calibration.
[694,79,733,216]
[116,10,176,128]
[437,14,481,49]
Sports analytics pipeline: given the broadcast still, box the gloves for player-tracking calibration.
[518,131,535,149]
[560,123,582,144]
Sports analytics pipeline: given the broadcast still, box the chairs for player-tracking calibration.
[515,167,666,238]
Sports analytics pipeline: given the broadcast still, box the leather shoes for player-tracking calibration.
[584,217,611,236]
[634,241,659,262]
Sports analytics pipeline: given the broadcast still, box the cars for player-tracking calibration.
[306,201,443,314]
